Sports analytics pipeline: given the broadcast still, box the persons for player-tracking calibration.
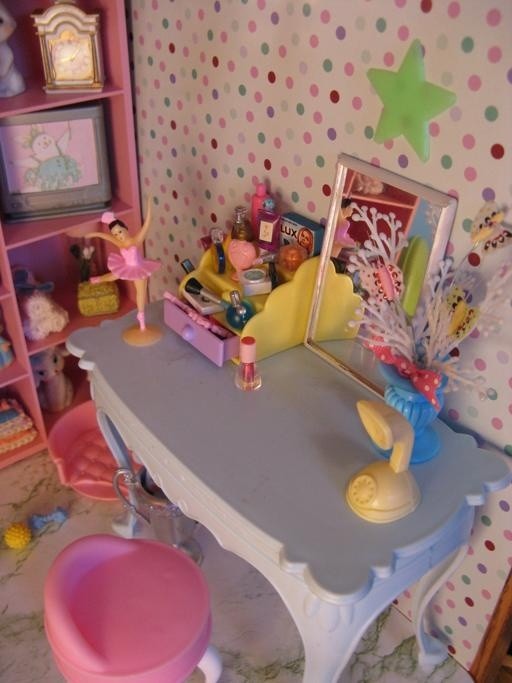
[84,191,161,332]
[0,0,30,99]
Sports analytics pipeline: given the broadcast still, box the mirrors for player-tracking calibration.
[303,154,457,402]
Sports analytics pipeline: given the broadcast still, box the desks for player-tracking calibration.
[64,294,511,683]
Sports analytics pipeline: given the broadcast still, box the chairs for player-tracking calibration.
[48,398,142,501]
[45,529,225,683]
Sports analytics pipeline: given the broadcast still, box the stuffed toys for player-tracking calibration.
[31,506,68,528]
[19,288,69,340]
[6,521,32,549]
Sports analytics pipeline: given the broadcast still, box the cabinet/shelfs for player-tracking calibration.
[0,0,148,468]
